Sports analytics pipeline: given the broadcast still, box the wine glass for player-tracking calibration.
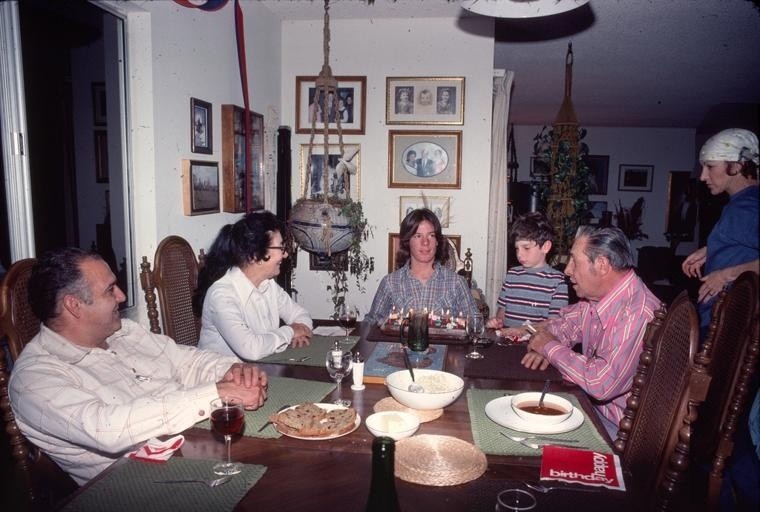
[464,313,485,359]
[207,397,246,476]
[338,303,358,345]
[325,350,354,408]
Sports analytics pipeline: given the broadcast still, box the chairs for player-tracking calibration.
[616,293,700,510]
[1,258,75,512]
[644,267,760,512]
[139,236,206,348]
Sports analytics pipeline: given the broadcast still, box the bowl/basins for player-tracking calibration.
[365,411,421,440]
[386,368,465,412]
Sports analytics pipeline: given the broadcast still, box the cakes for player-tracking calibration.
[383,314,469,341]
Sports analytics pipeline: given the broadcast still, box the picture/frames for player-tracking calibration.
[221,104,265,213]
[183,159,220,216]
[295,76,367,135]
[399,196,450,229]
[190,97,213,154]
[300,144,361,202]
[90,81,106,126]
[387,129,462,190]
[95,129,110,184]
[584,155,609,195]
[388,233,461,274]
[618,163,655,192]
[530,157,552,176]
[386,76,464,126]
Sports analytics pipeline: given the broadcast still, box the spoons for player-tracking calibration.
[403,348,424,393]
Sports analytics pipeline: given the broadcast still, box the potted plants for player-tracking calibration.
[283,193,375,324]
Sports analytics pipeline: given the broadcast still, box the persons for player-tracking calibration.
[363,208,481,326]
[192,214,312,363]
[484,212,569,337]
[521,225,667,441]
[7,248,268,489]
[682,128,759,512]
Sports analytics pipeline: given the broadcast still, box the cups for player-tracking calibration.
[400,310,430,351]
[494,490,539,512]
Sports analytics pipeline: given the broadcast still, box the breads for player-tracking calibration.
[266,402,360,439]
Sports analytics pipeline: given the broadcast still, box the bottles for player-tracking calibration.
[350,350,365,392]
[365,436,401,512]
[330,341,343,370]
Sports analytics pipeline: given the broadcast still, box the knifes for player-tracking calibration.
[258,406,289,432]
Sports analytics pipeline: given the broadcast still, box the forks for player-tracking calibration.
[500,429,589,451]
[266,355,312,362]
[519,476,602,496]
[151,476,233,489]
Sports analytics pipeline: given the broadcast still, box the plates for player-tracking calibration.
[273,402,361,441]
[511,392,574,425]
[484,394,585,436]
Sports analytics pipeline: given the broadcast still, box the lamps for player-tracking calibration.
[460,0,589,20]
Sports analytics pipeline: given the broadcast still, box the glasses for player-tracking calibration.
[266,244,285,254]
